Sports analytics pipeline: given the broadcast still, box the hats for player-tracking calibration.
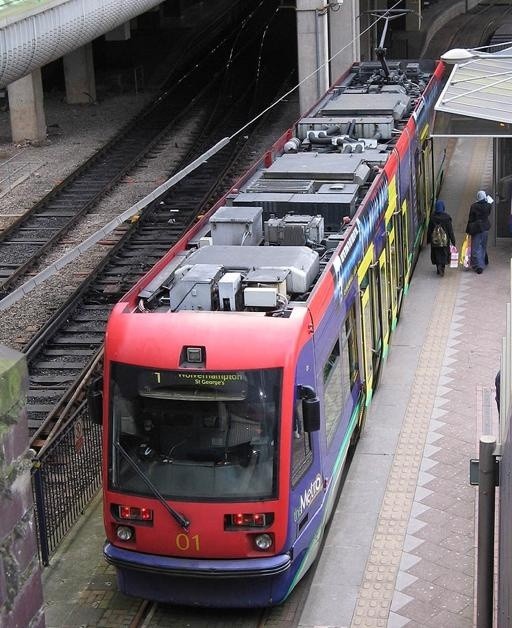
[477,190,487,200]
[435,201,444,213]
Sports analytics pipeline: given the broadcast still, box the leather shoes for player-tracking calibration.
[477,268,482,273]
[438,265,444,275]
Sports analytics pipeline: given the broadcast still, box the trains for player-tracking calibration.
[101,58,444,607]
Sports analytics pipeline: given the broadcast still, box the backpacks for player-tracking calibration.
[430,222,448,248]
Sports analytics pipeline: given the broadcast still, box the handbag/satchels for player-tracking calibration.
[460,232,472,268]
[448,245,459,268]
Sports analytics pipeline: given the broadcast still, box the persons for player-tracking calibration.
[425,200,458,275]
[463,190,492,274]
